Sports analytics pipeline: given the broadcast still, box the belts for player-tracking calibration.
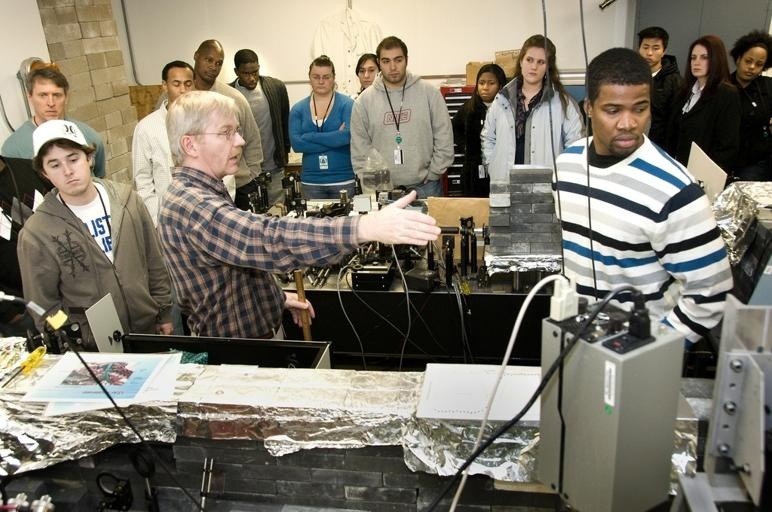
[255,325,282,339]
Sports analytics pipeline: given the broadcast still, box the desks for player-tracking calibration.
[238,194,570,369]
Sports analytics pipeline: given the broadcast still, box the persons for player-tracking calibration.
[153,90,442,340]
[452,64,508,198]
[17,118,175,353]
[2,67,106,181]
[133,38,291,229]
[289,34,454,204]
[551,47,735,353]
[636,28,772,183]
[480,34,585,183]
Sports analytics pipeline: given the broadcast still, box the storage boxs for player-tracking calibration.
[493,49,522,80]
[463,61,495,90]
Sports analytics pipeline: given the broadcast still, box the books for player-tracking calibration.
[21,351,184,415]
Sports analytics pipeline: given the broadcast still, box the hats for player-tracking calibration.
[32,119,88,157]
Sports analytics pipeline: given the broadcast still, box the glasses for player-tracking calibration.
[191,125,244,141]
